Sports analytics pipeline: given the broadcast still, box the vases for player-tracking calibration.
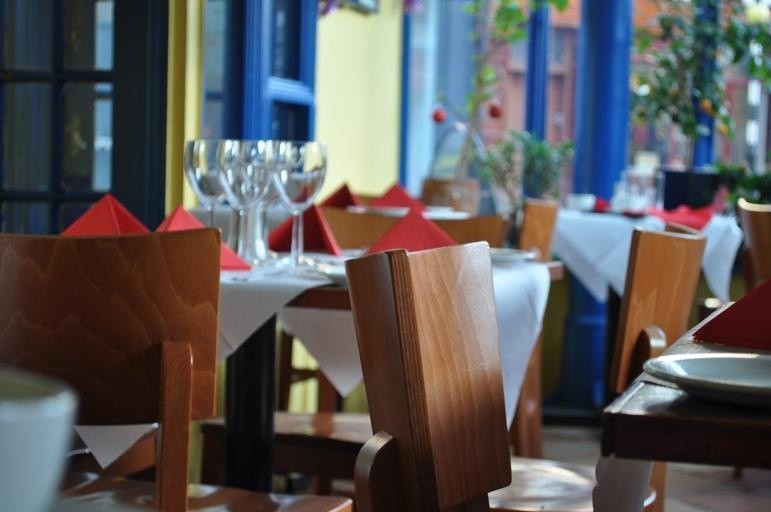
[653,165,724,220]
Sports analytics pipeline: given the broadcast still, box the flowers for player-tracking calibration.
[428,100,570,215]
[625,2,769,168]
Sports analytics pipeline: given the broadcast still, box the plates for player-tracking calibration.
[643,351,771,406]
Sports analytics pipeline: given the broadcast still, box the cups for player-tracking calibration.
[1,364,83,510]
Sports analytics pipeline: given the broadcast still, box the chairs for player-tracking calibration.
[1,179,771,508]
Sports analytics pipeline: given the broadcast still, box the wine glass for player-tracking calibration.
[188,139,329,277]
